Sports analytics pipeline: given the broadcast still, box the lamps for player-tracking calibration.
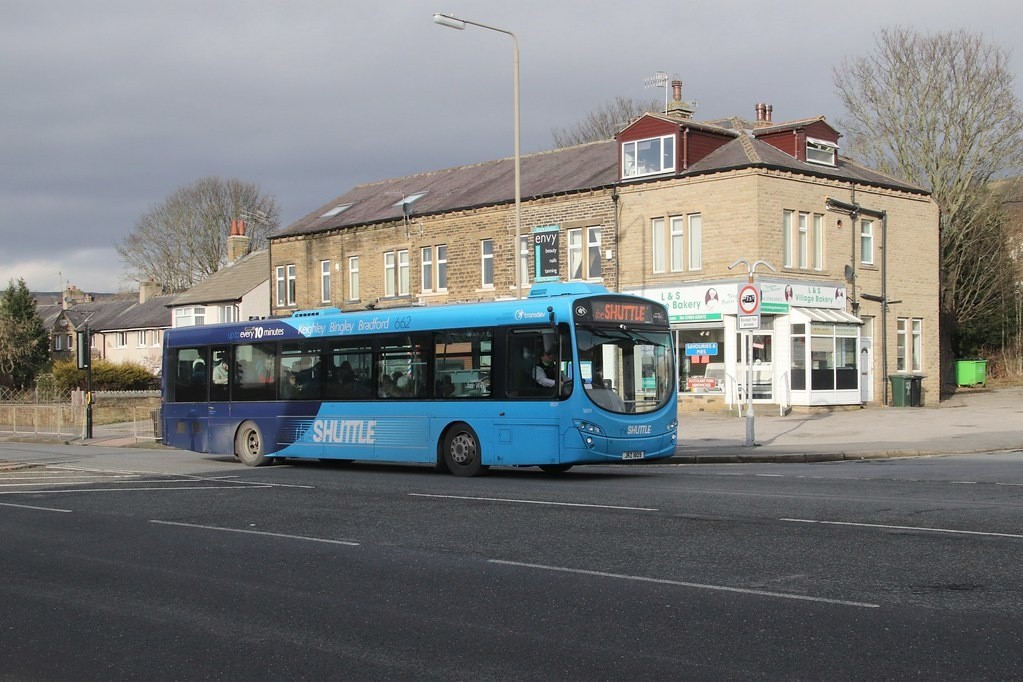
[845,296,859,310]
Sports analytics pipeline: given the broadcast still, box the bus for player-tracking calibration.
[162,283,681,479]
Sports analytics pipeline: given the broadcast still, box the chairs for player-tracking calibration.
[434,380,444,397]
[382,374,393,399]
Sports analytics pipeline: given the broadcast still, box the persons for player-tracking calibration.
[283,361,371,400]
[531,349,566,396]
[212,357,229,384]
[379,367,455,399]
[258,358,274,383]
[193,348,207,372]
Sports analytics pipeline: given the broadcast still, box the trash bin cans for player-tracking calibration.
[954,360,987,387]
[889,374,928,408]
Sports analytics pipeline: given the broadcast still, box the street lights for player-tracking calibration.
[432,12,524,296]
